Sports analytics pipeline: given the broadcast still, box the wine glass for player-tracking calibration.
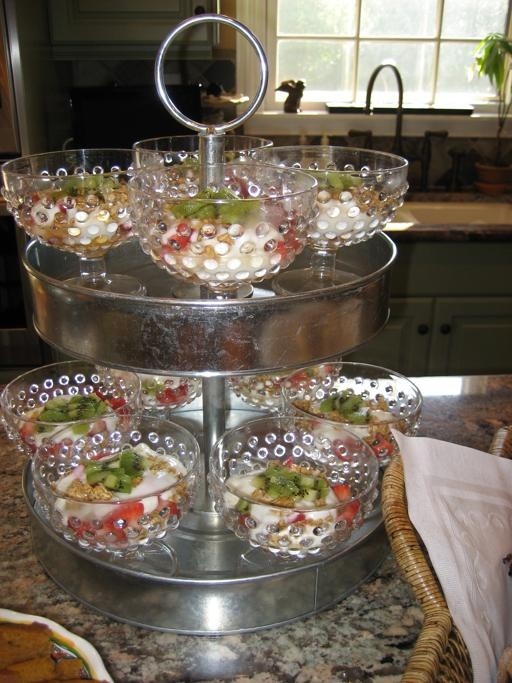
[285,360,417,481]
[0,148,162,299]
[210,416,381,571]
[249,144,409,297]
[132,135,272,176]
[132,161,320,303]
[32,414,203,578]
[226,360,342,432]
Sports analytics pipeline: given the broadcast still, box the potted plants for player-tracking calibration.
[471,30,512,197]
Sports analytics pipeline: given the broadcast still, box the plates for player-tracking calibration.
[1,609,114,682]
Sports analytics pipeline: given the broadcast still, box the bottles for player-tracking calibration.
[420,128,454,191]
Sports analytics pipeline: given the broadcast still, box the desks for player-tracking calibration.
[1,373,511,683]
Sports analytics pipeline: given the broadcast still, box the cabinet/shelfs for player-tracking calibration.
[20,12,398,636]
[343,244,512,375]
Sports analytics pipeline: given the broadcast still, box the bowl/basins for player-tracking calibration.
[0,359,204,455]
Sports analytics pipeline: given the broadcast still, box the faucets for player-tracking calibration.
[365,62,405,155]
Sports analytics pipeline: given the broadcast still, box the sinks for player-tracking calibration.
[402,189,481,204]
